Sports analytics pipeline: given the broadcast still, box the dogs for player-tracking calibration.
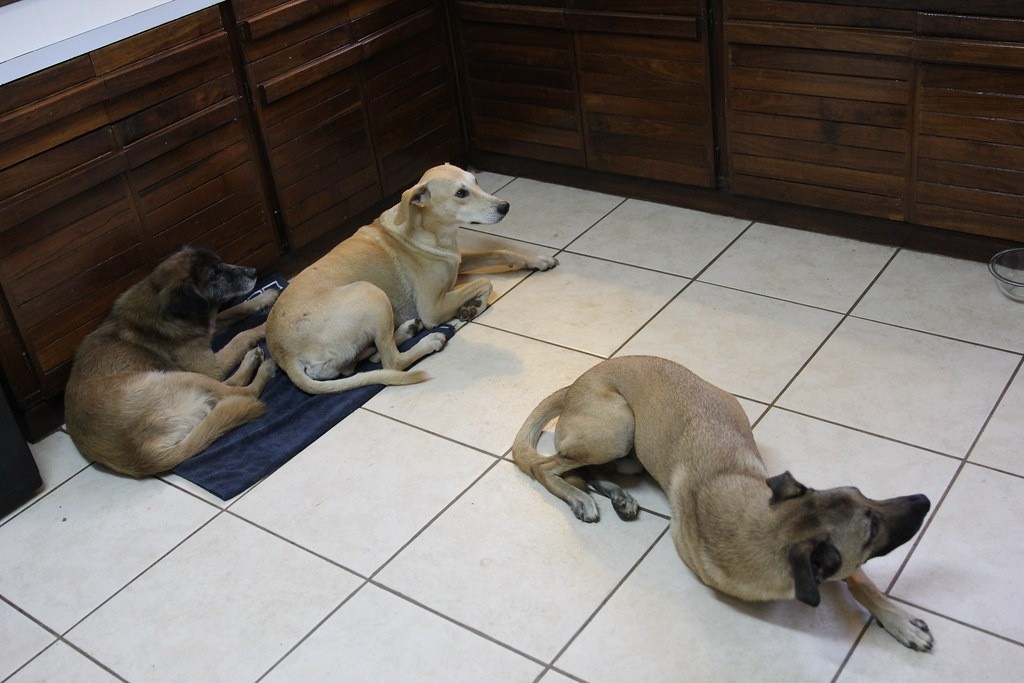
[63,242,281,480]
[513,355,934,653]
[264,163,559,395]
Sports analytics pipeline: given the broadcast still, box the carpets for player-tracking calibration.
[170,329,455,501]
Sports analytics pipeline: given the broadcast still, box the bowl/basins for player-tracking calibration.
[988,247,1024,303]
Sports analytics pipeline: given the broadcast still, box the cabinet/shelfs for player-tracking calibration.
[0,0,1024,445]
